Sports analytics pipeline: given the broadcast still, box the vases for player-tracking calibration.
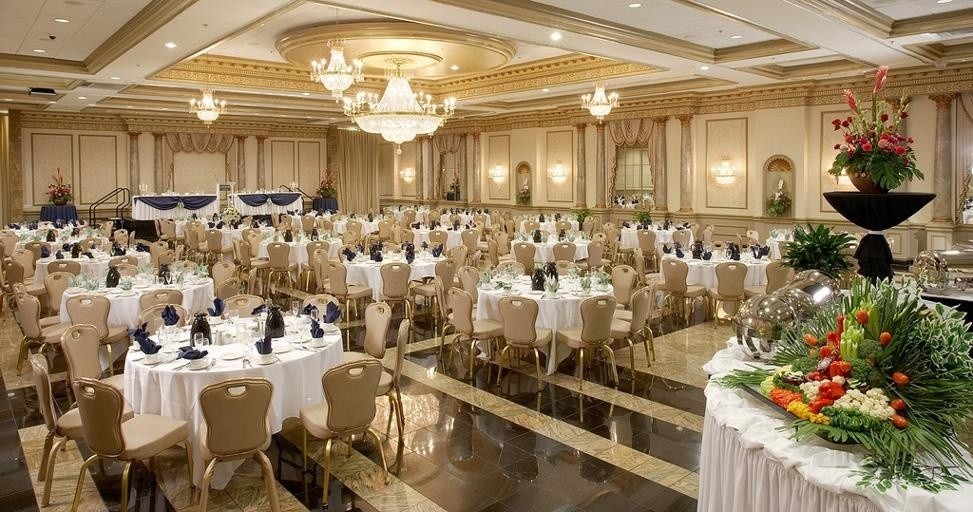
[51,198,67,207]
[321,193,331,198]
[844,166,899,193]
[774,205,785,217]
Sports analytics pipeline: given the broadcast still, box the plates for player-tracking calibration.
[65,274,210,297]
[347,254,441,264]
[480,275,610,299]
[128,319,339,371]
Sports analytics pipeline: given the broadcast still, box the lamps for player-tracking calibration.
[548,162,570,184]
[310,8,366,104]
[187,52,226,130]
[709,157,739,185]
[344,60,455,156]
[488,165,507,184]
[400,167,416,183]
[582,56,620,125]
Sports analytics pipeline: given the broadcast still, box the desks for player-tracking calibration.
[132,196,218,220]
[313,198,338,214]
[227,193,304,216]
[697,335,972,511]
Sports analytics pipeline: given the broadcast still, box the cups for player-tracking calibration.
[69,283,183,295]
[482,283,608,298]
[132,313,334,368]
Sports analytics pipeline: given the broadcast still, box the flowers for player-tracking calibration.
[720,280,973,493]
[47,168,75,202]
[827,64,924,189]
[316,169,337,197]
[766,181,791,217]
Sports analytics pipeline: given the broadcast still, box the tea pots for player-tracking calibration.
[428,220,460,231]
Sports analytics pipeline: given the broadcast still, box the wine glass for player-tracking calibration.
[156,272,172,287]
[492,263,596,294]
[514,231,591,243]
[299,230,317,242]
[99,244,134,257]
[722,247,749,260]
[154,299,319,353]
